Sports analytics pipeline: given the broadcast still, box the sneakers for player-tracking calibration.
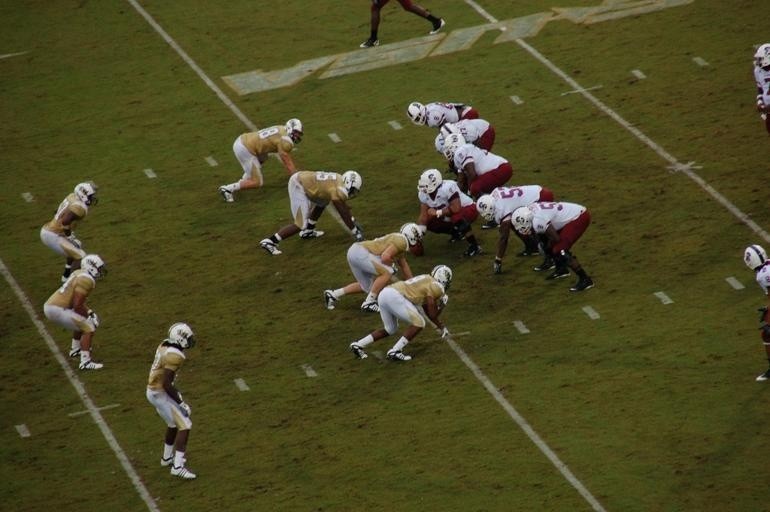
[219,185,234,203]
[361,300,380,313]
[350,342,368,359]
[79,360,103,370]
[324,290,337,309]
[171,465,197,479]
[360,39,380,47]
[69,346,92,357]
[161,456,187,466]
[429,18,446,34]
[386,348,412,361]
[464,245,483,257]
[299,230,325,238]
[516,250,594,291]
[756,368,770,383]
[449,232,465,243]
[259,238,282,255]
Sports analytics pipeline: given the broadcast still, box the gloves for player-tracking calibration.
[439,293,449,305]
[352,229,364,241]
[758,324,770,338]
[87,308,97,319]
[440,327,450,343]
[757,306,768,323]
[757,100,766,113]
[68,235,81,248]
[87,315,99,328]
[178,401,192,417]
[494,259,502,274]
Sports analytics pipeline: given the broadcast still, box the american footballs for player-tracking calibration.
[410,243,424,255]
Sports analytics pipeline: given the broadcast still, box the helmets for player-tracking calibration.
[81,254,107,278]
[168,322,196,348]
[753,43,770,68]
[343,170,362,198]
[285,118,303,143]
[744,244,768,271]
[400,222,423,246]
[406,101,532,237]
[75,183,98,206]
[431,264,453,290]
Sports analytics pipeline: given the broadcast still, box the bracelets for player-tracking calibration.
[435,208,443,218]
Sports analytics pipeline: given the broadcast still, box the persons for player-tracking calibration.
[744,244,770,382]
[323,221,424,313]
[476,185,555,276]
[42,254,109,370]
[417,169,484,259]
[260,170,364,257]
[348,264,454,363]
[39,179,97,283]
[442,134,514,230]
[751,42,770,133]
[359,0,446,49]
[146,321,198,480]
[406,102,480,173]
[433,119,496,181]
[510,200,595,292]
[217,118,304,205]
